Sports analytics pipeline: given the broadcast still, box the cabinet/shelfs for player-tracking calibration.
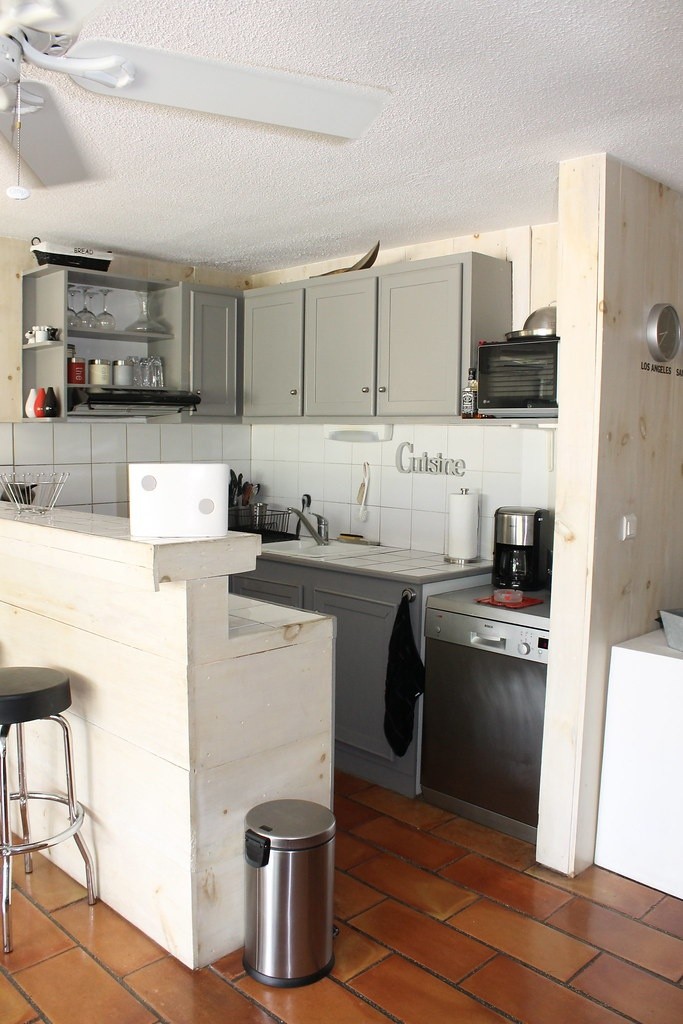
[229,557,419,764]
[20,252,513,421]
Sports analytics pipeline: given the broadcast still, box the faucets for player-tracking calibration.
[287,507,329,546]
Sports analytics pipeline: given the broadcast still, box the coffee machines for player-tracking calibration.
[491,505,550,591]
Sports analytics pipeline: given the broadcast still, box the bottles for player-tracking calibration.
[25,387,58,418]
[124,290,167,334]
[461,368,481,419]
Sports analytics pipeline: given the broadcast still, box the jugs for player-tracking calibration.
[499,545,537,582]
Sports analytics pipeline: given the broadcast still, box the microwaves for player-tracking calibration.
[477,340,559,418]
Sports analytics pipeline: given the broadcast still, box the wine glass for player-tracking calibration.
[67,284,118,330]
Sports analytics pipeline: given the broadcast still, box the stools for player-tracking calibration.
[0,669,97,953]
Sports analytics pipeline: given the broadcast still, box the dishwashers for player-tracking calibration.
[419,583,553,845]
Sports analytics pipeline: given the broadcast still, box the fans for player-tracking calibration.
[1,0,393,182]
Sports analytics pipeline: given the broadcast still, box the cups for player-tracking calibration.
[67,343,164,387]
[36,331,48,342]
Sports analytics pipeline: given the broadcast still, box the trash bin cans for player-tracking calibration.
[242,799,338,988]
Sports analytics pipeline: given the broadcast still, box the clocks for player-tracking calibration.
[647,303,682,363]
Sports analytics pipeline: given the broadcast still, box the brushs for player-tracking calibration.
[359,462,369,523]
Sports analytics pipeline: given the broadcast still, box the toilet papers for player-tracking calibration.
[448,494,478,560]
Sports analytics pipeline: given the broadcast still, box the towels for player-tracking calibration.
[383,594,424,757]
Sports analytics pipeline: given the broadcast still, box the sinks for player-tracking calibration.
[262,542,368,555]
